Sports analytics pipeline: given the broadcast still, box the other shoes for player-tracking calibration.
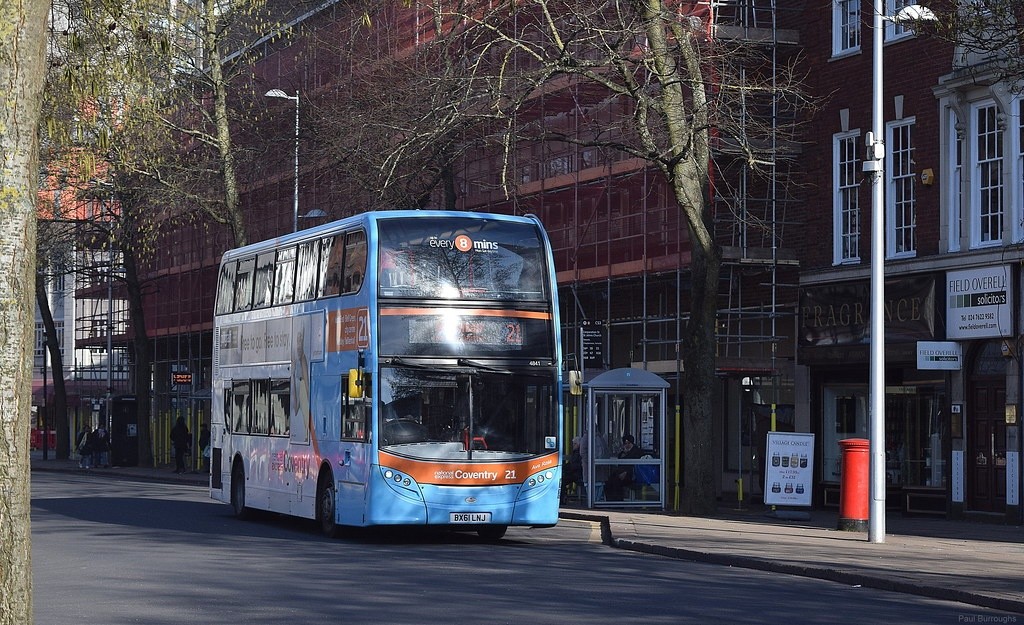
[104,464,110,468]
[78,463,83,469]
[86,465,90,469]
[179,472,187,475]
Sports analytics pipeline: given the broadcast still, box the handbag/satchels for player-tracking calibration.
[203,441,210,458]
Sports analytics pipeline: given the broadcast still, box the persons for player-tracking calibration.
[603,435,642,502]
[559,436,583,505]
[76,423,112,470]
[199,424,210,473]
[579,422,608,503]
[169,415,189,474]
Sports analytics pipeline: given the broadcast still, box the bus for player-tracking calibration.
[209,209,584,542]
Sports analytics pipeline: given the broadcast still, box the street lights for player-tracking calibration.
[87,179,116,433]
[868,5,941,545]
[265,88,299,232]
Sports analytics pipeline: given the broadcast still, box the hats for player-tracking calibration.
[199,423,208,427]
